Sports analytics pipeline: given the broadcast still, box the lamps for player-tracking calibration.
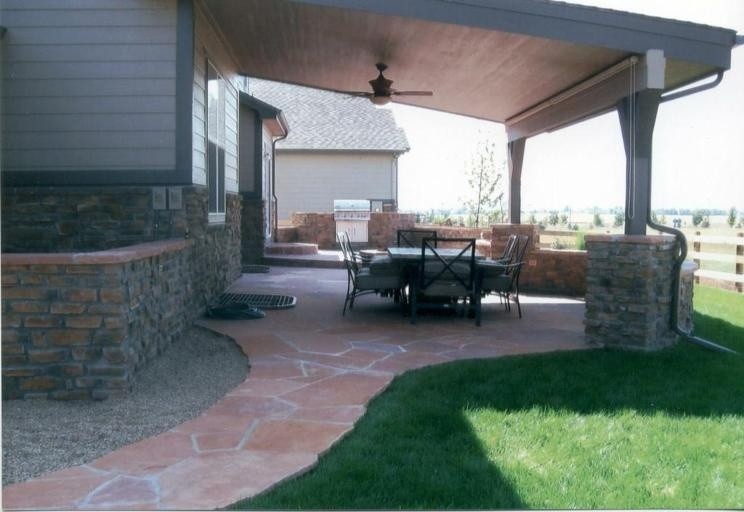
[369,95,390,105]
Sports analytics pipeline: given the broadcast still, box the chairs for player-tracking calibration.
[332,230,532,326]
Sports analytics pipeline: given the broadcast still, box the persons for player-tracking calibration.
[672,218,677,228]
[677,218,682,227]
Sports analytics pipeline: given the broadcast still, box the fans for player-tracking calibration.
[338,62,436,97]
